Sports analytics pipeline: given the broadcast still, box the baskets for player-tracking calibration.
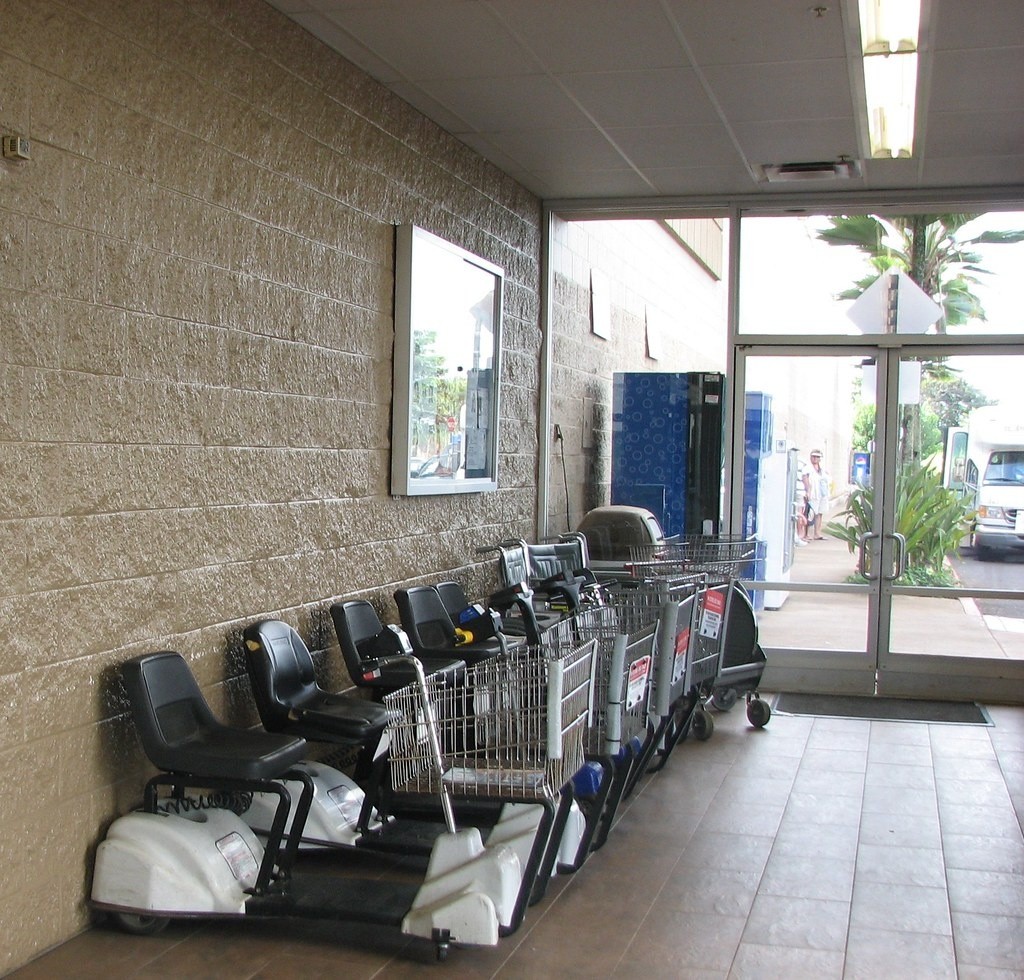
[381,535,757,807]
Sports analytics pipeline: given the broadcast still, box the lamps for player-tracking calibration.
[839,0,936,160]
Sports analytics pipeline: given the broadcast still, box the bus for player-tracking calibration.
[969,439,1024,562]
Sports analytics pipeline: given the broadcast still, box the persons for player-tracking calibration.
[802,450,822,540]
[807,452,829,540]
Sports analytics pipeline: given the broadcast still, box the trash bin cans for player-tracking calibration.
[575,505,666,562]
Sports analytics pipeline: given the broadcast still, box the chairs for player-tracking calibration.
[436,581,526,647]
[242,619,389,738]
[329,597,467,690]
[554,534,585,572]
[395,585,517,663]
[529,541,562,577]
[125,650,308,783]
[482,539,549,609]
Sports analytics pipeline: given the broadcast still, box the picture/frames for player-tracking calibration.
[395,222,498,492]
